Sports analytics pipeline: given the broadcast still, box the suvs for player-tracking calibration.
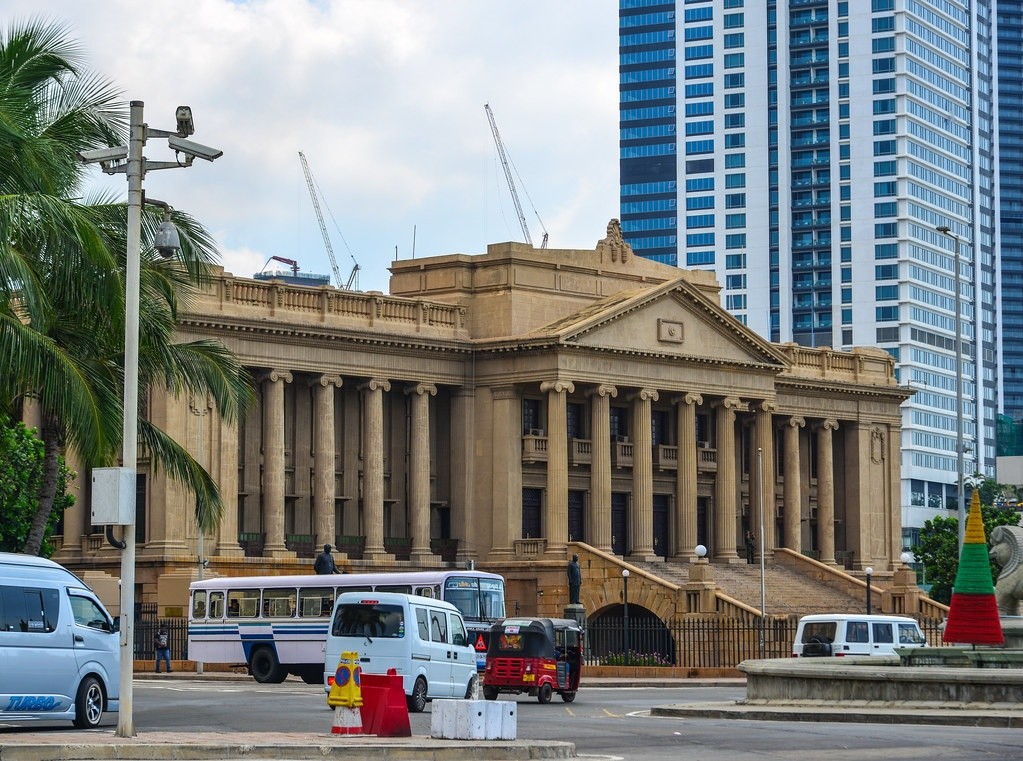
[792,613,930,658]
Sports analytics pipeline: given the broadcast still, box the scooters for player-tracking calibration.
[482,617,584,705]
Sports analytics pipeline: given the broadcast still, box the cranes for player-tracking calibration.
[485,104,548,249]
[299,151,362,291]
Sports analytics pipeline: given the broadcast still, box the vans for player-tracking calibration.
[324,592,478,713]
[0,552,120,730]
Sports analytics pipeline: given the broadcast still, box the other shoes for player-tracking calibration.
[156,670,161,673]
[167,670,173,672]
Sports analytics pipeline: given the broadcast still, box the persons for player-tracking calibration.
[155,625,172,672]
[567,554,582,603]
[194,602,205,617]
[230,603,238,616]
[314,544,339,574]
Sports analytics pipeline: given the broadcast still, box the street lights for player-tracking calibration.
[622,569,630,665]
[865,567,874,615]
[936,226,966,559]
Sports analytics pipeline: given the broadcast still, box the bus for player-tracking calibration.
[187,571,506,684]
